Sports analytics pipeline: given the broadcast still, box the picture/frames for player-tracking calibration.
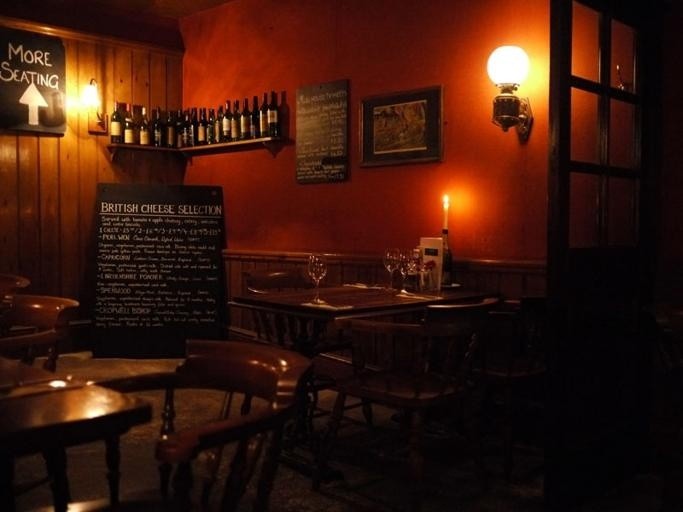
[359,84,444,169]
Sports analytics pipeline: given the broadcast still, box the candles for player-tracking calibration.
[444,194,449,229]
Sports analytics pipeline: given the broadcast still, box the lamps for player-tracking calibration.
[486,44,533,143]
[82,76,108,135]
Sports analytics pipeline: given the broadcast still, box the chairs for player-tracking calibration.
[426,296,499,320]
[307,314,438,451]
[0,293,80,373]
[39,336,300,512]
[240,259,310,287]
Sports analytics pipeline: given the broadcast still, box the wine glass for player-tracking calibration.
[306,255,328,305]
[399,248,411,294]
[406,248,424,293]
[383,248,400,293]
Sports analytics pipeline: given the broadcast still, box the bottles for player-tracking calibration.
[176,110,183,148]
[266,91,279,137]
[259,93,268,138]
[190,108,197,147]
[110,102,122,143]
[139,108,150,145]
[440,229,452,285]
[165,111,174,146]
[215,106,224,144]
[278,90,288,135]
[184,112,189,147]
[239,98,249,141]
[222,101,232,142]
[206,109,215,145]
[153,107,164,146]
[124,104,134,144]
[197,109,206,146]
[250,96,259,140]
[230,100,240,142]
